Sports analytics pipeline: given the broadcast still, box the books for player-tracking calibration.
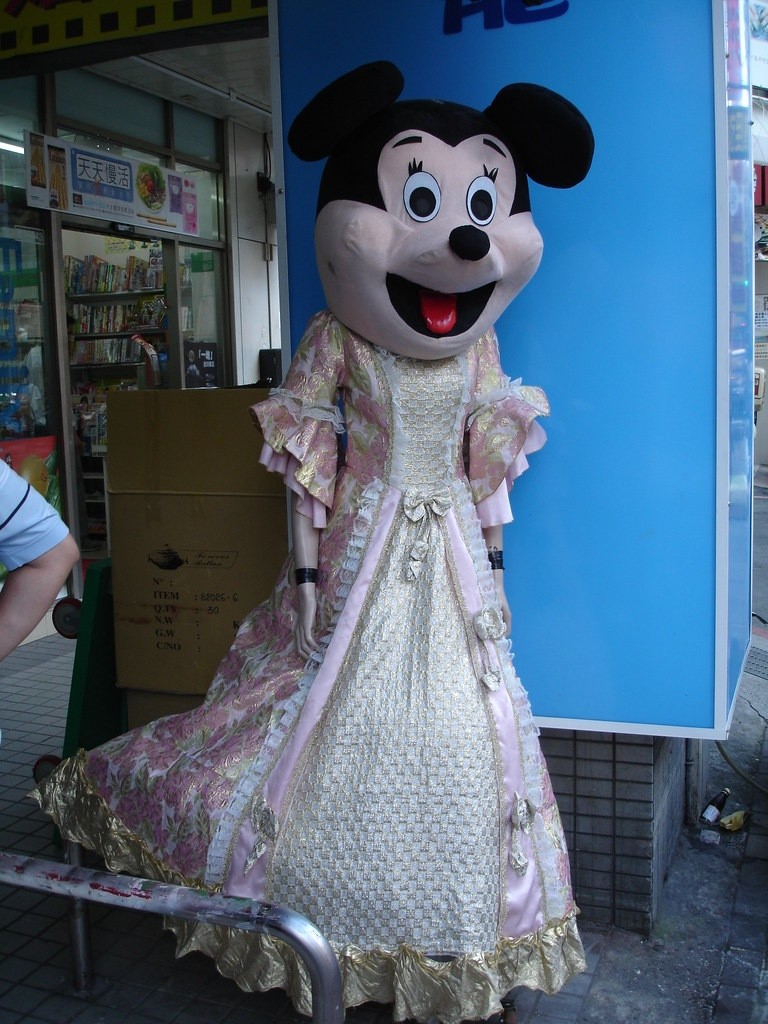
[63,255,149,365]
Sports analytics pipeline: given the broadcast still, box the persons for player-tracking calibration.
[23,314,75,437]
[0,458,79,661]
[20,365,41,436]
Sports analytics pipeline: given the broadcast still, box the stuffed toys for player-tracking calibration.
[25,60,594,1024]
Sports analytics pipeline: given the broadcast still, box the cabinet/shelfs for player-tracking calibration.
[64,286,193,559]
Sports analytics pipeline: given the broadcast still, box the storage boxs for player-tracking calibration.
[127,687,204,730]
[103,389,288,696]
